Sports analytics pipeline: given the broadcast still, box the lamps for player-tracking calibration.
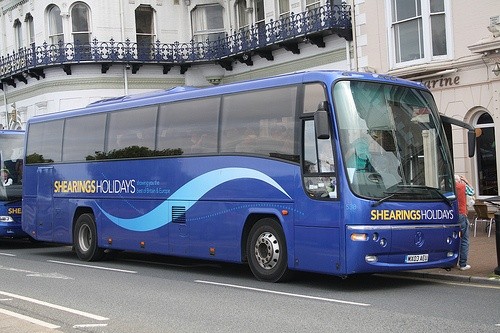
[492,62,500,76]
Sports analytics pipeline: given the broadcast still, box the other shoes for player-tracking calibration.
[459,264,471,271]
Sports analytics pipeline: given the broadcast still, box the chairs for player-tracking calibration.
[473,205,495,238]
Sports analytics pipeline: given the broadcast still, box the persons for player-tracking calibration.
[441,174,476,270]
[134,123,355,166]
[14,155,23,183]
[0,167,13,186]
[2,141,13,160]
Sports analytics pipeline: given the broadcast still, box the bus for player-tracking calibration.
[0,69,476,283]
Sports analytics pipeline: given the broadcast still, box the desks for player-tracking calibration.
[478,195,500,210]
